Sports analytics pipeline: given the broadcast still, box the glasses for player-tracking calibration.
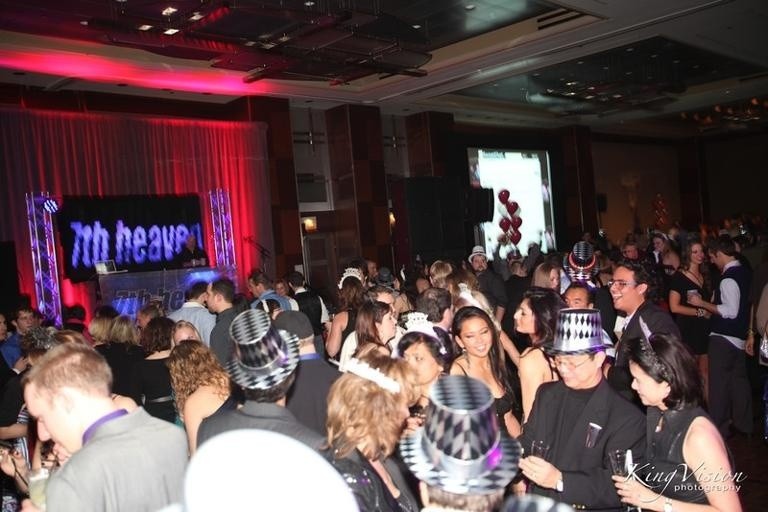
[607,278,638,288]
[552,354,595,370]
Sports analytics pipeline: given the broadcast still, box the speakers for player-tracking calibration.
[597,195,607,214]
[470,188,496,223]
[403,176,470,264]
[0,240,22,319]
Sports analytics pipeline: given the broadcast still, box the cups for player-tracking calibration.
[28,467,51,510]
[687,290,698,298]
[329,314,335,322]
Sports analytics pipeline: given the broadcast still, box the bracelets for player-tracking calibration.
[746,327,757,337]
[696,307,705,318]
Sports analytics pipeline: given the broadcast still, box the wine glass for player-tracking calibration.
[532,442,548,459]
[614,449,629,477]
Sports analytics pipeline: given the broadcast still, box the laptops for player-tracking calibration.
[93,259,129,275]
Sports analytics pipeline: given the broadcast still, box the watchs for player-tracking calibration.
[662,495,674,512]
[556,473,567,492]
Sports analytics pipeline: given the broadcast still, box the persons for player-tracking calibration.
[90,294,179,413]
[231,261,298,316]
[521,234,612,312]
[20,344,188,512]
[402,255,455,419]
[604,263,683,412]
[318,376,409,512]
[746,258,768,443]
[331,258,402,366]
[179,275,231,451]
[492,235,521,367]
[195,309,324,454]
[275,310,335,436]
[455,242,491,323]
[452,306,522,438]
[686,237,755,442]
[612,225,685,277]
[284,269,329,362]
[170,236,209,267]
[611,336,742,512]
[509,307,647,511]
[515,287,568,422]
[668,237,710,410]
[0,298,56,512]
[56,294,91,345]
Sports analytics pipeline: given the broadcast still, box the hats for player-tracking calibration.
[375,266,394,287]
[540,302,613,352]
[567,240,599,280]
[220,308,300,392]
[468,245,489,264]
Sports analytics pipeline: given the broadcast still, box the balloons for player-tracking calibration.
[497,188,522,246]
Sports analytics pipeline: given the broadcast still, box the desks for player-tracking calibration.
[86,266,216,318]
